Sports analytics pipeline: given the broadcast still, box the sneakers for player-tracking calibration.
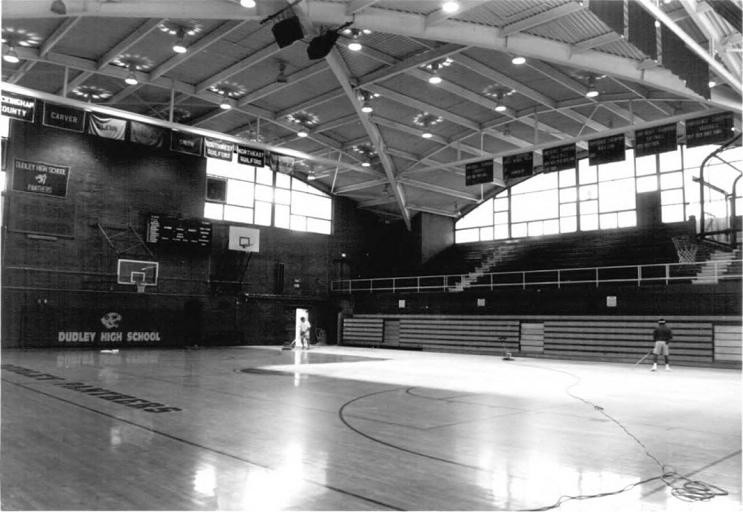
[651,368,672,372]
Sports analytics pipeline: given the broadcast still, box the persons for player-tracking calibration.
[649,318,673,371]
[298,316,311,349]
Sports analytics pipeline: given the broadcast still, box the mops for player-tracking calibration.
[281,336,303,350]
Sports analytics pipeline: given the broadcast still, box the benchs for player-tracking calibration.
[417,218,742,286]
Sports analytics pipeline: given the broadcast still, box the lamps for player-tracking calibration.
[297,119,308,138]
[421,116,432,138]
[4,40,19,63]
[349,30,361,51]
[307,25,341,60]
[173,28,186,53]
[512,56,526,65]
[125,62,138,84]
[220,90,230,109]
[361,92,373,112]
[361,148,371,167]
[428,62,441,84]
[494,90,506,112]
[587,74,598,98]
[306,168,314,180]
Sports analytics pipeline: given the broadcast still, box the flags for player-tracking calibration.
[167,129,202,158]
[85,112,127,143]
[202,137,234,163]
[129,120,164,149]
[10,157,71,201]
[41,100,86,134]
[235,144,265,167]
[267,151,295,175]
[0,89,37,124]
